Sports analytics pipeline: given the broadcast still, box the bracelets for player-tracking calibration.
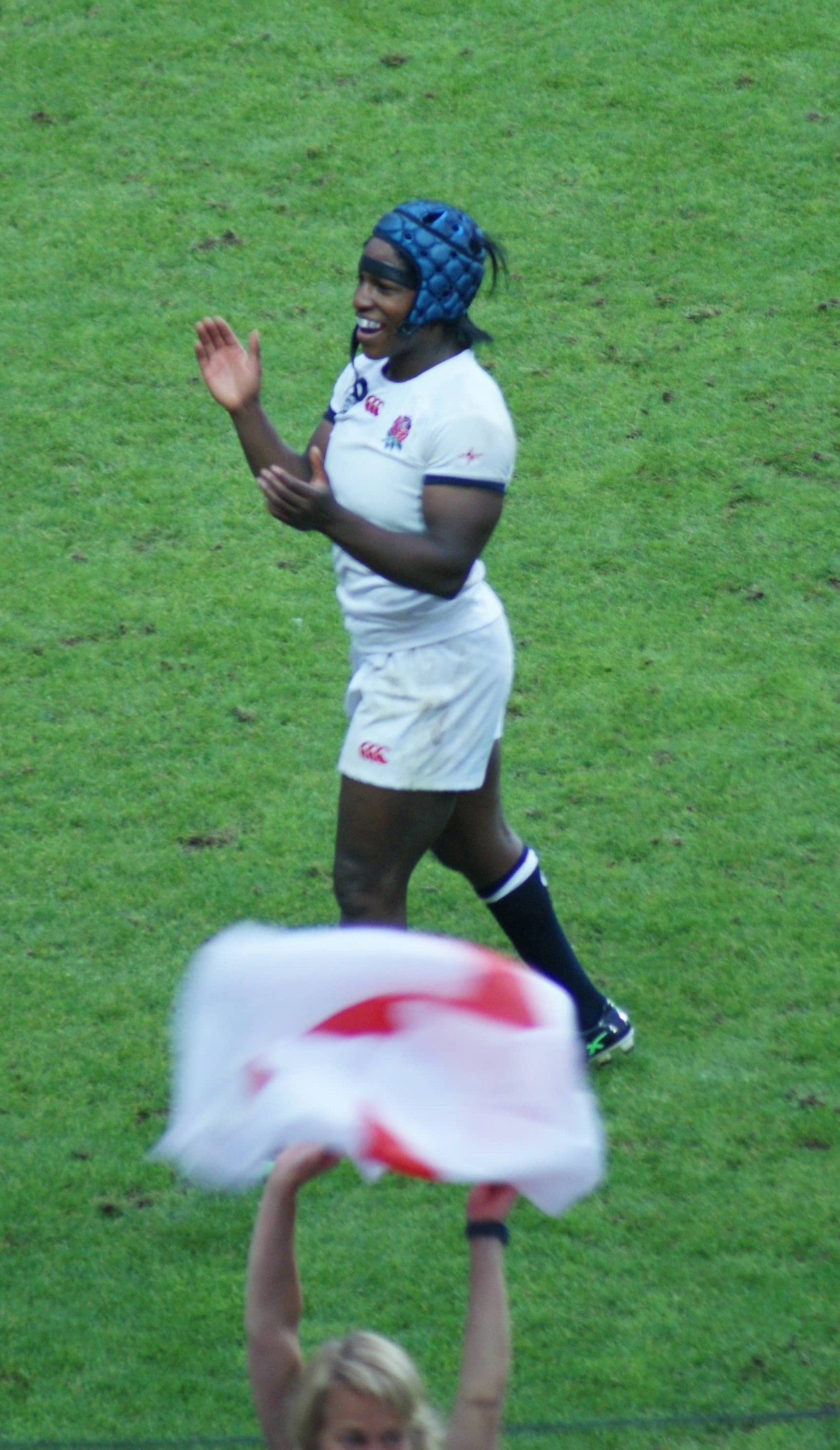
[464,1217,512,1248]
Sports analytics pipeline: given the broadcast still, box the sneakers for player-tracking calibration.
[581,998,635,1067]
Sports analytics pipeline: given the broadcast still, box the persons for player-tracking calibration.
[191,194,641,1075]
[242,1132,520,1450]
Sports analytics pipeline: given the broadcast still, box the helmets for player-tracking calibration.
[372,198,486,342]
